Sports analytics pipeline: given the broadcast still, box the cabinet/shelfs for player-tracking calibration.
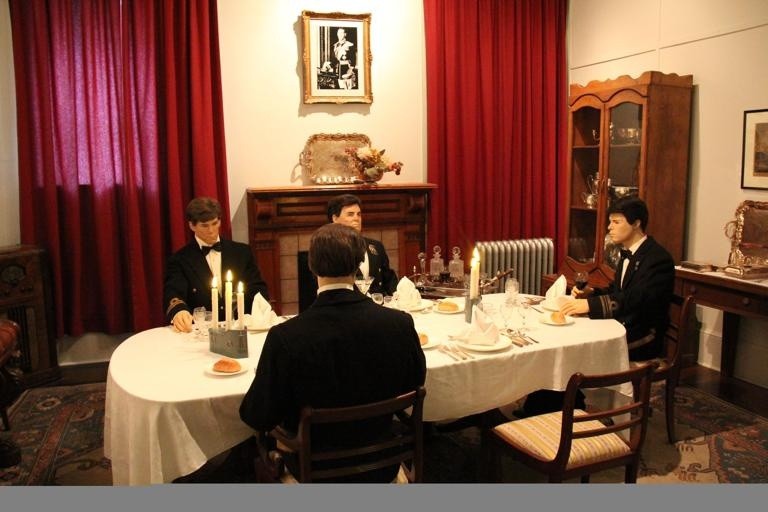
[564,70,693,287]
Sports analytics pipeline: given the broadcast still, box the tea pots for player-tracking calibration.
[580,172,610,208]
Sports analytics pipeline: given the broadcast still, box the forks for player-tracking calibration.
[440,343,474,362]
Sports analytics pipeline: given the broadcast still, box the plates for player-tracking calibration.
[414,328,439,350]
[206,361,246,376]
[540,313,575,326]
[246,315,286,333]
[412,298,433,311]
[456,332,511,351]
[540,300,560,311]
[435,301,465,314]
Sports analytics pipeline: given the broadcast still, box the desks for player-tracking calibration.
[673,265,768,378]
[419,274,500,298]
[0,247,59,389]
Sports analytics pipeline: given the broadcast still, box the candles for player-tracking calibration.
[211,275,219,327]
[236,281,246,330]
[469,257,478,299]
[224,269,233,330]
[472,246,480,295]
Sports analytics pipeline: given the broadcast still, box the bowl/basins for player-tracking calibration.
[611,186,638,200]
[618,128,641,139]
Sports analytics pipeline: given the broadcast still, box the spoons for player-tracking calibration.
[503,329,540,347]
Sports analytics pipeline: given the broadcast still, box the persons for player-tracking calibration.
[511,194,676,420]
[163,197,270,334]
[326,193,400,297]
[321,27,357,90]
[170,221,428,485]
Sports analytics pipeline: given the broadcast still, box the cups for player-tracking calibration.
[505,278,519,304]
[193,307,212,338]
[372,293,393,308]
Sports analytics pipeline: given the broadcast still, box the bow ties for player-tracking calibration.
[202,242,222,257]
[620,248,633,261]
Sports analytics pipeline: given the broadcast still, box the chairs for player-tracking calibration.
[252,386,426,484]
[488,359,660,483]
[629,294,694,444]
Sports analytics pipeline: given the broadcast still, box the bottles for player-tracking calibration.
[429,245,464,277]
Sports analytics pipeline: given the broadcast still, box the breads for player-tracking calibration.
[550,312,566,324]
[213,356,241,373]
[419,333,428,345]
[437,300,459,312]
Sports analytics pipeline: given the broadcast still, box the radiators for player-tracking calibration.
[474,237,554,297]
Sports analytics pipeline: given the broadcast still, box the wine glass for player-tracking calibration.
[482,303,530,331]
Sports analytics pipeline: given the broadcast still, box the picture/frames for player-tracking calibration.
[302,10,373,103]
[740,109,768,189]
